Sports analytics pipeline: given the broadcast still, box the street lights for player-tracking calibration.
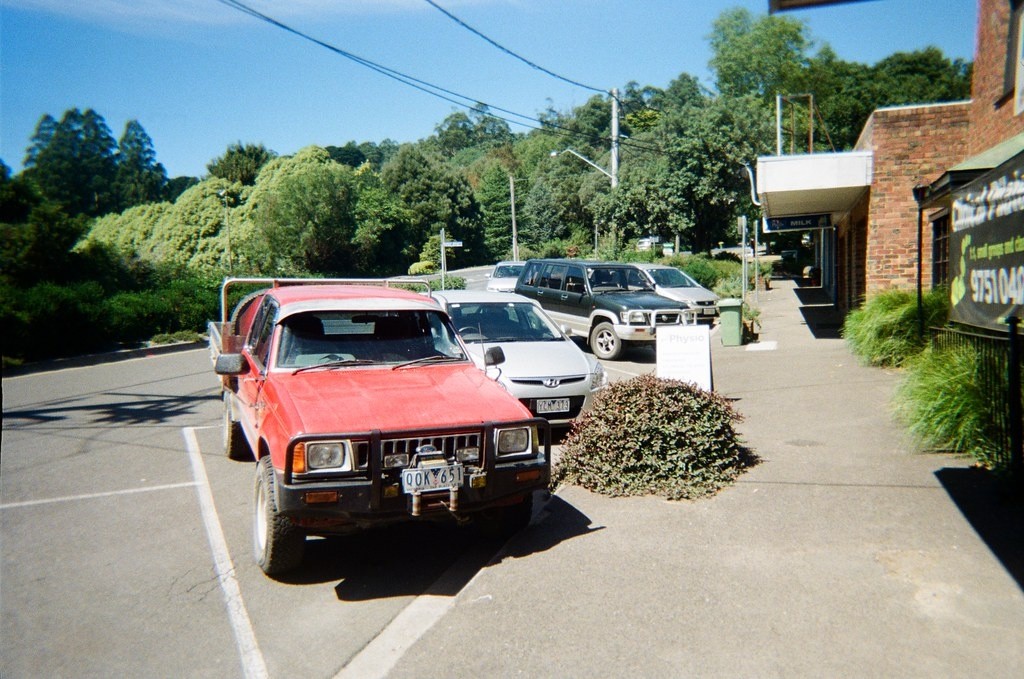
[219,188,234,275]
[551,149,621,187]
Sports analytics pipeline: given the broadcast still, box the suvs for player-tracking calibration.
[514,258,702,359]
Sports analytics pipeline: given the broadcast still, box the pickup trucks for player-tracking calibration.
[208,276,553,576]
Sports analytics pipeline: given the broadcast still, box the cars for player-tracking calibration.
[418,290,608,429]
[487,260,526,294]
[628,262,721,324]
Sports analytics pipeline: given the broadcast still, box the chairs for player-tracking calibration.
[487,308,510,330]
[373,316,416,342]
[288,316,325,341]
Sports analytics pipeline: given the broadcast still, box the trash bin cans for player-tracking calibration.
[717,298,744,346]
